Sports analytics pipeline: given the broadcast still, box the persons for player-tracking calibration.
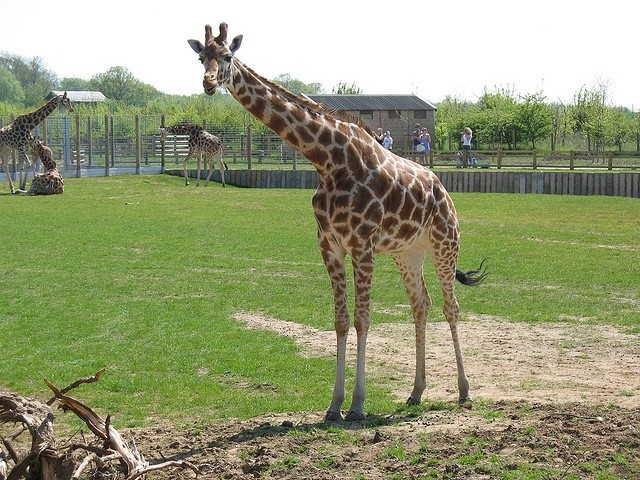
[460,127,474,167]
[411,123,424,165]
[417,128,431,165]
[371,128,384,150]
[381,130,393,153]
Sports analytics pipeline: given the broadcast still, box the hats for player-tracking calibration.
[384,130,391,135]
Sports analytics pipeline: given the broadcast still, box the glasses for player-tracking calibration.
[415,126,418,128]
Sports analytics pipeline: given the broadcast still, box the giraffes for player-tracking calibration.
[0,90,75,195]
[187,22,492,423]
[158,122,228,188]
[15,137,65,195]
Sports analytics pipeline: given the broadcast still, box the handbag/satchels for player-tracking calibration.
[416,143,425,151]
